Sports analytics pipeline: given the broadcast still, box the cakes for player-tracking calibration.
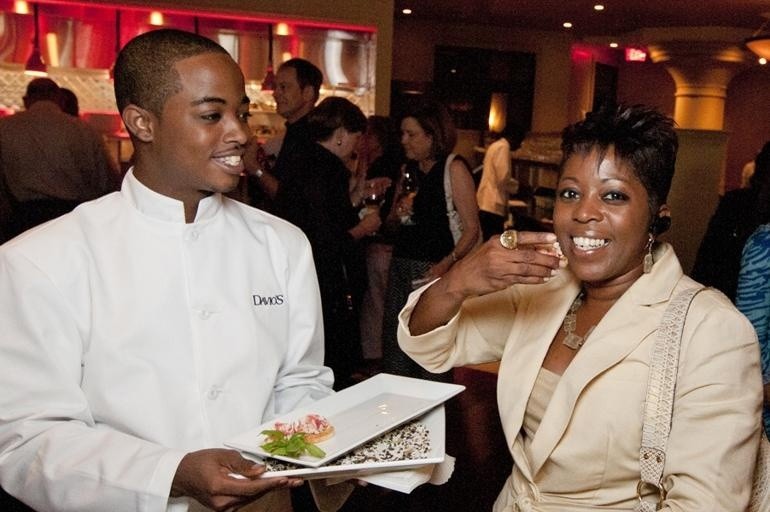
[273,414,335,443]
[534,240,563,258]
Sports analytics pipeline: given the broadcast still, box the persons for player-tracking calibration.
[395,101,765,512]
[1,27,373,511]
[1,56,770,468]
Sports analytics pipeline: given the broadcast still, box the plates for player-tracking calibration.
[227,402,445,483]
[224,368,467,468]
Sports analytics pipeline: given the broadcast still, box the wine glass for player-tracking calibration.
[362,186,384,239]
[395,169,420,226]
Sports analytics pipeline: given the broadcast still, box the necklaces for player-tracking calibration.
[563,291,600,349]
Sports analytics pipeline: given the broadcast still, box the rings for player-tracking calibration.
[500,228,519,250]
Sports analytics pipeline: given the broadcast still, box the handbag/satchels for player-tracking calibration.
[444,153,483,247]
